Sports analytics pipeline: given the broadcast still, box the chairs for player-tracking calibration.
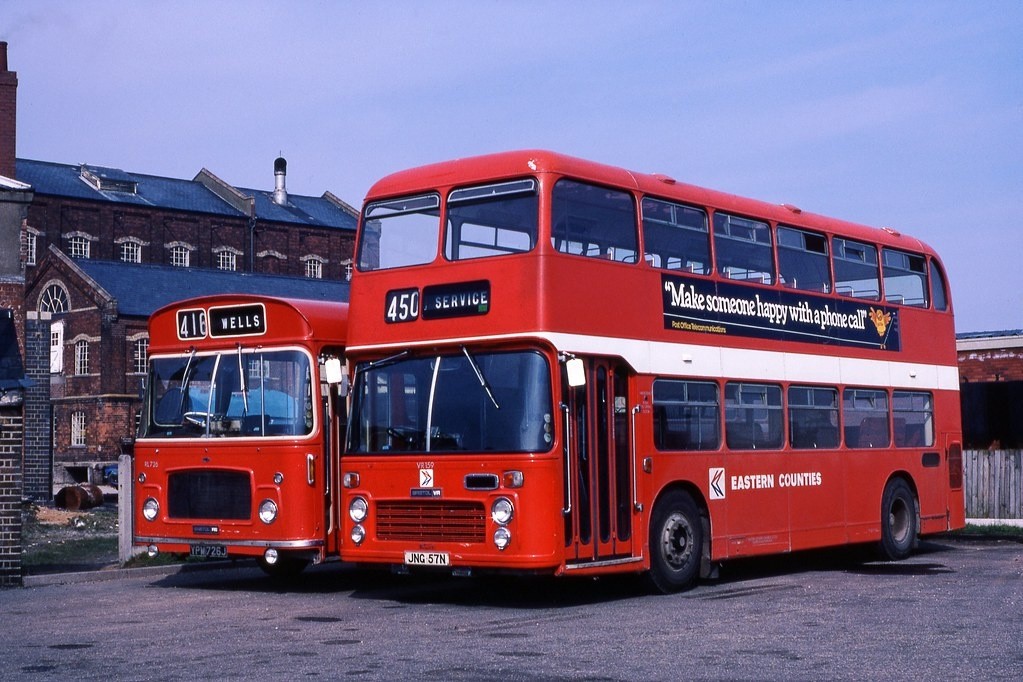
[580,247,613,261]
[662,260,694,272]
[622,253,655,267]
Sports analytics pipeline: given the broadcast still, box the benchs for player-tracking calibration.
[826,286,854,298]
[904,298,928,308]
[764,276,797,289]
[851,290,879,301]
[694,265,731,279]
[885,294,905,305]
[730,271,764,284]
[798,281,826,294]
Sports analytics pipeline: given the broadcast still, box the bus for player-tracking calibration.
[130,294,348,582]
[339,147,964,594]
